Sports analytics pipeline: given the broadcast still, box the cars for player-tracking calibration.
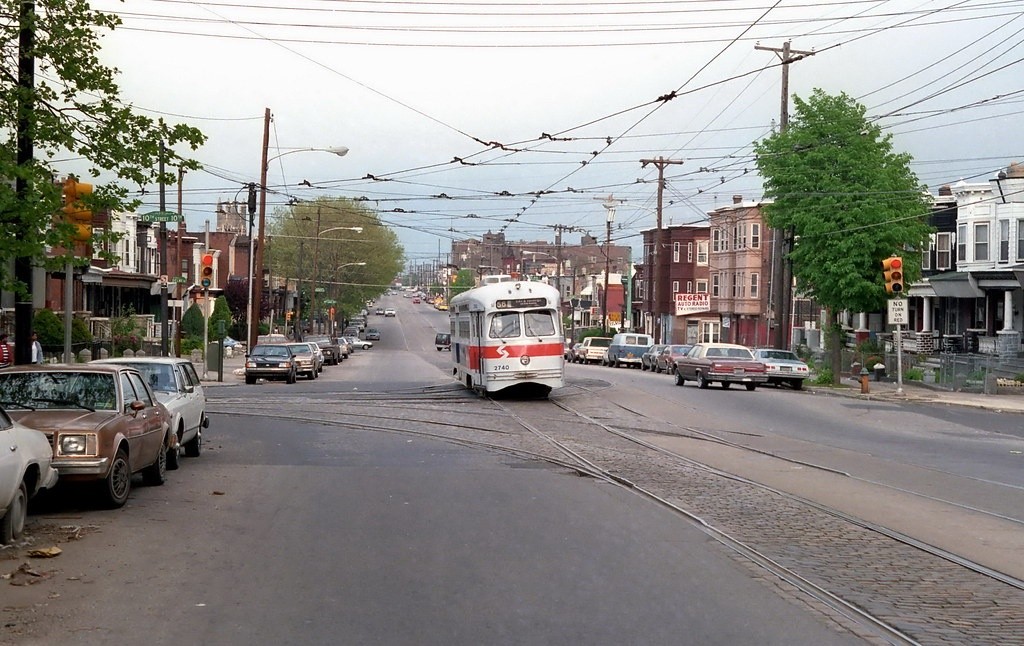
[342,287,449,338]
[0,406,61,545]
[304,334,373,366]
[365,328,380,341]
[68,356,211,470]
[672,342,770,391]
[0,364,173,510]
[244,343,297,384]
[568,336,810,390]
[270,341,325,380]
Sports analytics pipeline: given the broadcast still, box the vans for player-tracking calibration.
[607,334,654,369]
[436,334,452,351]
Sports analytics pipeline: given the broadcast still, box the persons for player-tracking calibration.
[289,326,294,335]
[31,330,44,364]
[0,333,13,369]
[273,327,279,334]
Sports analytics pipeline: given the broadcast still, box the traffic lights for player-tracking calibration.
[62,179,93,241]
[201,252,213,287]
[881,258,904,293]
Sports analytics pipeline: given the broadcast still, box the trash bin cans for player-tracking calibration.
[207,342,219,372]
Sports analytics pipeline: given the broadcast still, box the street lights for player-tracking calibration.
[521,251,560,291]
[604,203,663,344]
[247,142,370,350]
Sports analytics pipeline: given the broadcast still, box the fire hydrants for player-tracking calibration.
[856,367,869,394]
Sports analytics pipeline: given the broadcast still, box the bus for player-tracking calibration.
[448,274,566,398]
